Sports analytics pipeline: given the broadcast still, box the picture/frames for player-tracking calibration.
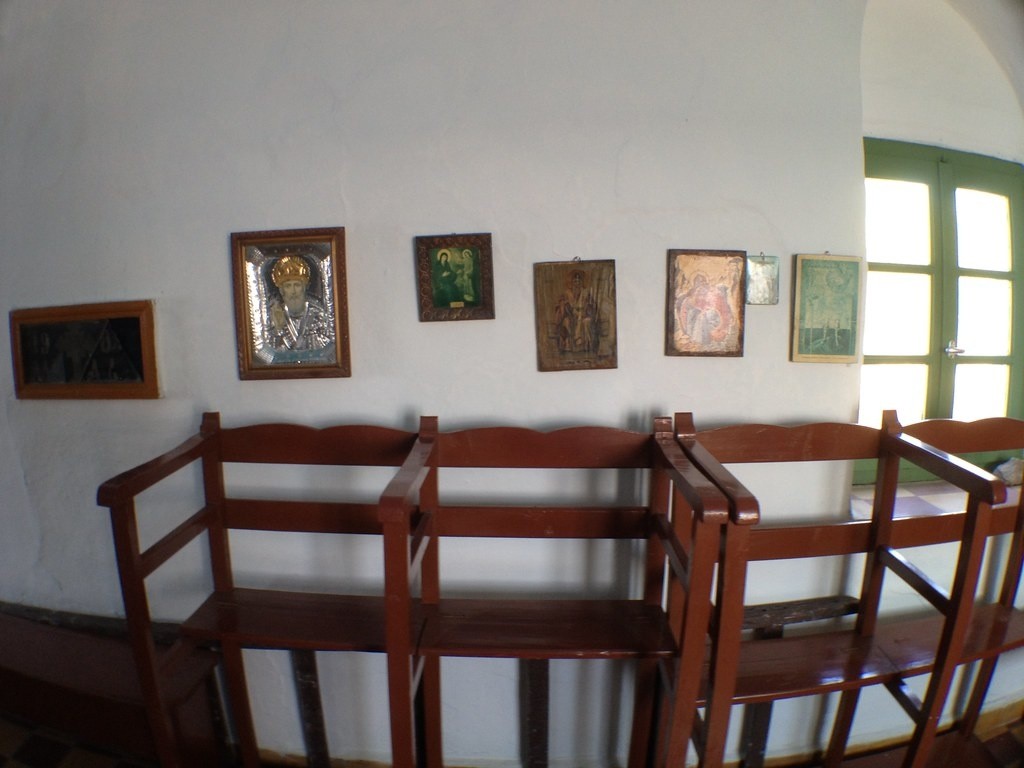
[666,247,746,358]
[534,259,617,371]
[9,300,162,399]
[412,232,494,322]
[746,254,779,305]
[231,226,352,383]
[790,254,863,365]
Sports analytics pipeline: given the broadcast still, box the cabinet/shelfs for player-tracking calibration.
[94,411,1023,767]
